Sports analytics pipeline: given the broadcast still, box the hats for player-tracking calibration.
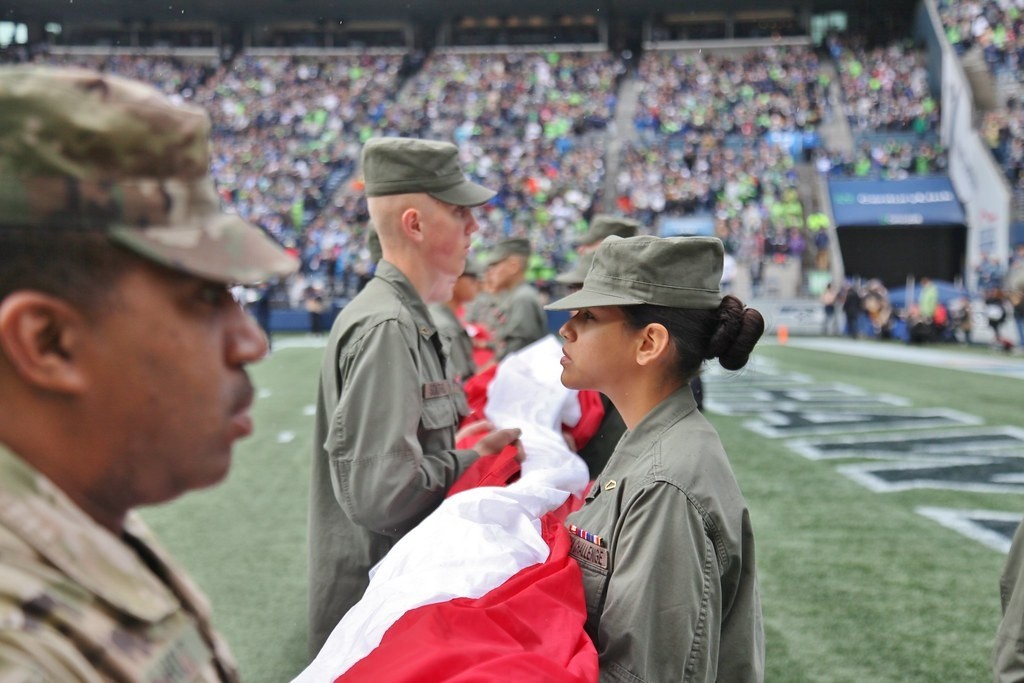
[0,64,303,285]
[361,137,498,207]
[487,236,532,265]
[555,248,595,284]
[463,259,478,275]
[543,236,724,311]
[571,215,643,245]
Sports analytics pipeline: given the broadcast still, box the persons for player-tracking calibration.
[1,0,1024,482]
[542,233,764,683]
[308,136,526,665]
[990,515,1023,682]
[0,57,302,683]
